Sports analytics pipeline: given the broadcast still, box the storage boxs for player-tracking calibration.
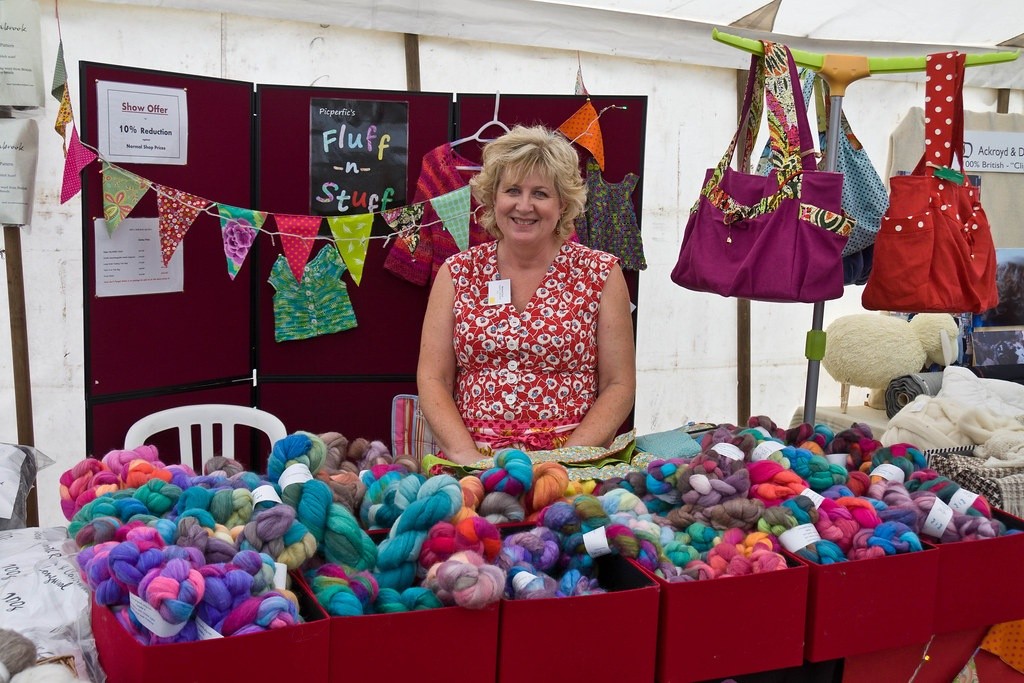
[90,507,1024,683]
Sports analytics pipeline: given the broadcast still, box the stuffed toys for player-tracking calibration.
[823,312,959,416]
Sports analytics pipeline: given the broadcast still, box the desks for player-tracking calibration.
[788,404,890,441]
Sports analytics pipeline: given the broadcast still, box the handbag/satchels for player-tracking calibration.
[670,38,845,302]
[861,52,1001,313]
[798,56,890,284]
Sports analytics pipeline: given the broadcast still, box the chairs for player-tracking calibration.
[123,404,288,476]
[391,392,446,461]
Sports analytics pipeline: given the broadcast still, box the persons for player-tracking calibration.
[417,124,637,471]
